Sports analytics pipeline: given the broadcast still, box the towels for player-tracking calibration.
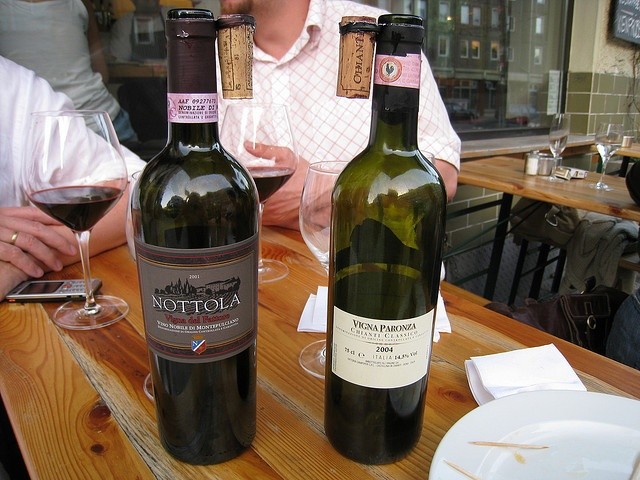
[464,343,587,407]
[296,286,453,343]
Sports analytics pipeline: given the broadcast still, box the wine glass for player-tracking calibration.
[219,98,298,288]
[547,112,573,181]
[586,121,622,194]
[22,107,131,333]
[299,161,357,384]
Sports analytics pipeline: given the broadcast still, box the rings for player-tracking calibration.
[10,231,18,245]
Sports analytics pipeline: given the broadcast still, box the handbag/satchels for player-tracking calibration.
[510,196,581,245]
[556,293,611,356]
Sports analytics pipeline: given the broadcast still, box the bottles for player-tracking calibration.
[130,9,261,466]
[323,14,448,466]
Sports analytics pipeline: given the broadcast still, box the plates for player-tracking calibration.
[426,390,638,475]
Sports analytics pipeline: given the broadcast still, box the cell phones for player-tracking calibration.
[4,279,103,303]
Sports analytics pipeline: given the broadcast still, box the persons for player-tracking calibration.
[104,1,175,66]
[215,0,462,231]
[1,1,140,145]
[2,55,148,301]
